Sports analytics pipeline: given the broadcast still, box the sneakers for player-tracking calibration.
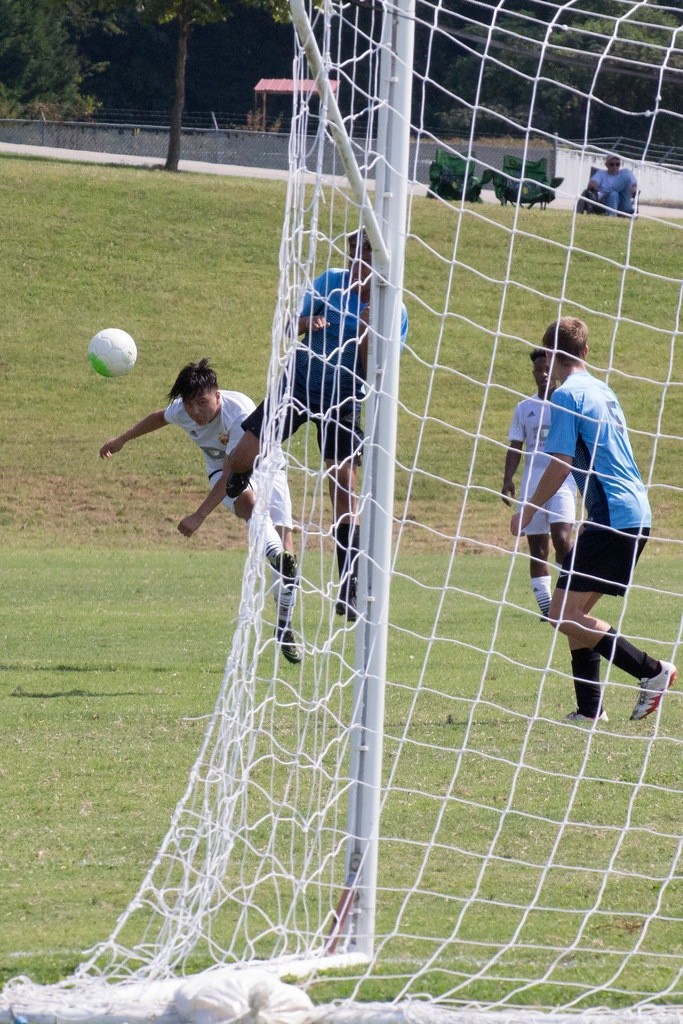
[336,597,356,622]
[274,625,302,664]
[629,660,678,720]
[226,469,253,498]
[276,551,299,591]
[565,708,609,722]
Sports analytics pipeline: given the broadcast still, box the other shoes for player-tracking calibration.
[540,614,548,622]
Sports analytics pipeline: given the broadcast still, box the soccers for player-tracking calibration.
[86,328,138,378]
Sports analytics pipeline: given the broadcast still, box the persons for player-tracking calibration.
[502,350,578,620]
[508,316,677,725]
[100,358,305,663]
[224,228,410,621]
[585,154,638,216]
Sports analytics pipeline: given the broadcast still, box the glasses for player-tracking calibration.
[608,162,620,167]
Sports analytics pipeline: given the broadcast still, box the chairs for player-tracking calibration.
[426,148,494,203]
[493,155,565,210]
[584,166,641,219]
